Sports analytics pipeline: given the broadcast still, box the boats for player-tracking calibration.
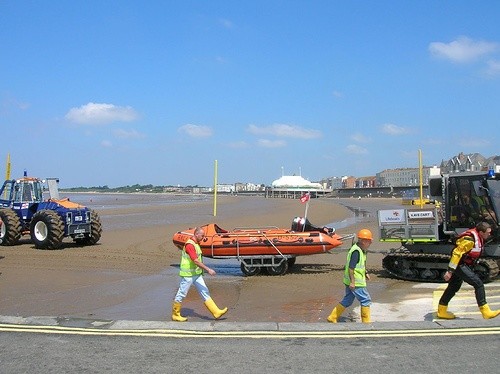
[172,189,345,256]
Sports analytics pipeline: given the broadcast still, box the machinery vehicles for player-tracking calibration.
[0,169,102,251]
[378,149,500,284]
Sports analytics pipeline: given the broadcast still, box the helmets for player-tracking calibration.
[357,228,374,240]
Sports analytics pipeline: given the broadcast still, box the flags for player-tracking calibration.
[300,194,308,205]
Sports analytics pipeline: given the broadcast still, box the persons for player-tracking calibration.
[472,191,497,224]
[437,222,500,319]
[172,227,227,321]
[23,185,32,201]
[359,195,361,200]
[327,229,374,323]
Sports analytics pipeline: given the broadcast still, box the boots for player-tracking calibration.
[361,306,371,323]
[203,297,228,319]
[171,303,188,322]
[437,305,456,319]
[327,303,346,323]
[479,303,500,319]
